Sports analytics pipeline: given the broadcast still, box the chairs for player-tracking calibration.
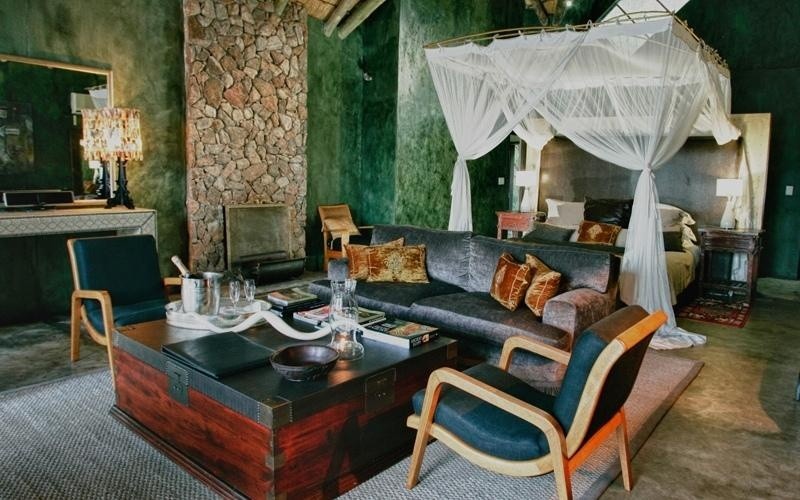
[61,234,204,393]
[402,303,670,500]
[317,203,374,274]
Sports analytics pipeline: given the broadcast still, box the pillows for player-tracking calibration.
[682,236,695,248]
[488,251,533,313]
[523,255,564,319]
[661,223,698,241]
[582,194,635,227]
[656,202,696,225]
[544,198,583,227]
[324,216,362,239]
[364,244,430,285]
[661,230,683,254]
[342,236,407,286]
[521,218,576,242]
[576,219,622,250]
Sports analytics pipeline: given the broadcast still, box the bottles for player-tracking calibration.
[170,255,201,280]
[327,276,365,360]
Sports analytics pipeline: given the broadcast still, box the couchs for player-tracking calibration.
[304,223,623,400]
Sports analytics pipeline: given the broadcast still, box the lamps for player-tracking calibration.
[513,169,540,211]
[79,105,107,198]
[102,107,144,210]
[714,178,745,229]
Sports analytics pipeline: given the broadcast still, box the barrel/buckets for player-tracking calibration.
[181,271,221,317]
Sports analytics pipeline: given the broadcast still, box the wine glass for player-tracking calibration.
[226,279,242,316]
[244,279,257,311]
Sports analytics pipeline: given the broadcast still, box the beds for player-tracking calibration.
[519,196,700,316]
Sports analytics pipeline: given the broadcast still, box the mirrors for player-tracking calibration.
[0,54,115,207]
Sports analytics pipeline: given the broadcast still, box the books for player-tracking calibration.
[291,301,442,350]
[265,286,319,306]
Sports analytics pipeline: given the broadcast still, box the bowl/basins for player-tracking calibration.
[270,344,342,383]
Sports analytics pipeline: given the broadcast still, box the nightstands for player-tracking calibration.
[496,210,536,240]
[701,226,767,308]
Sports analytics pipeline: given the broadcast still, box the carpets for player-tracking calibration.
[1,295,710,500]
[672,281,755,330]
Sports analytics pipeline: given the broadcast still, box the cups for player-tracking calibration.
[179,271,224,317]
